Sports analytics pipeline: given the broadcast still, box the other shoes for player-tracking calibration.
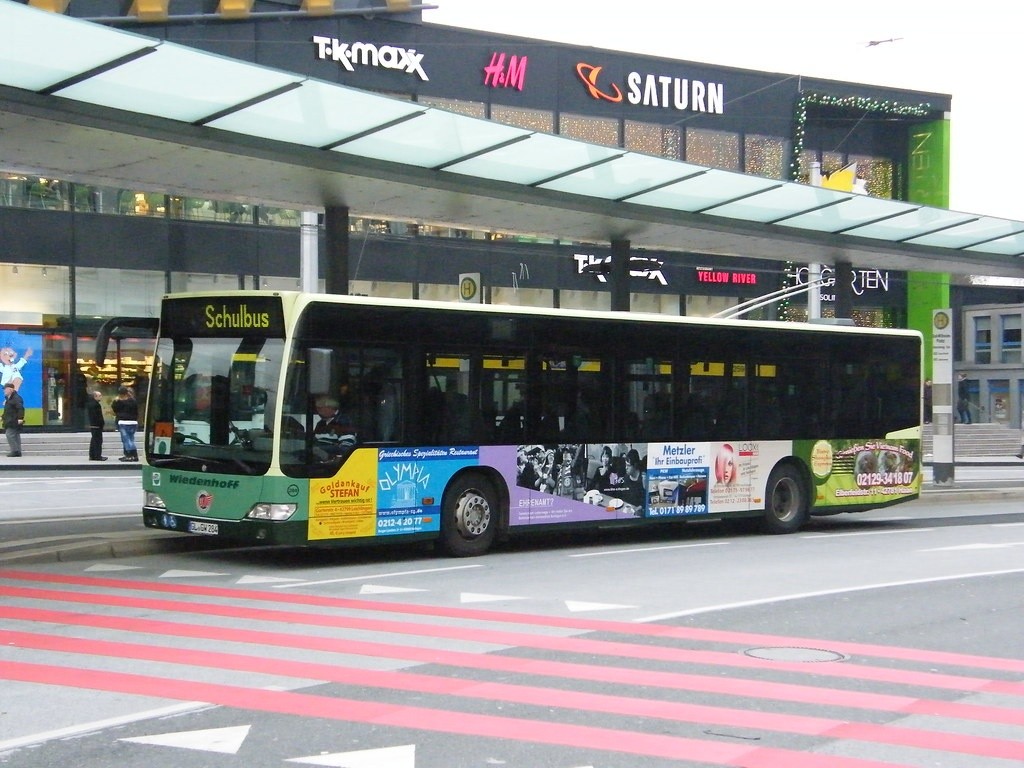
[925,421,929,423]
[7,451,22,457]
[1016,454,1023,458]
[90,457,108,461]
[959,420,972,424]
[119,456,139,462]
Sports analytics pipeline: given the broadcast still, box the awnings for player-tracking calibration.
[0,0,1024,280]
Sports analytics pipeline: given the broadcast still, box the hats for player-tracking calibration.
[544,449,554,458]
[118,387,127,394]
[2,383,14,389]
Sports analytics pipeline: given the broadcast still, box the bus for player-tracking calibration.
[96,288,925,558]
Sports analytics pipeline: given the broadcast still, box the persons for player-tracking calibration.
[88,391,108,461]
[517,445,580,496]
[957,373,971,423]
[583,443,647,516]
[2,383,25,457]
[313,394,356,463]
[712,444,737,497]
[924,378,932,424]
[1016,411,1024,459]
[111,386,139,462]
